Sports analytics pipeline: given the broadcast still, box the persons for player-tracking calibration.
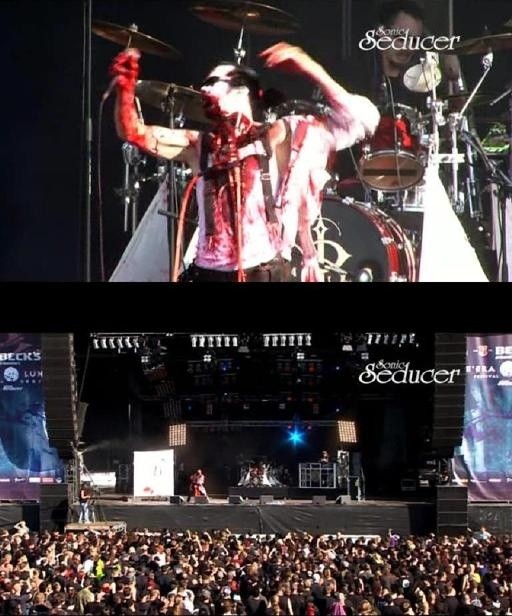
[110,40,382,283]
[77,482,92,523]
[2,520,512,616]
[326,0,424,207]
[186,468,209,503]
[318,450,330,483]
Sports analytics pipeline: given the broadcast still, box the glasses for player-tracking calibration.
[203,74,231,86]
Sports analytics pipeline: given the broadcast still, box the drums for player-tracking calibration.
[264,98,338,188]
[286,195,416,285]
[355,102,425,192]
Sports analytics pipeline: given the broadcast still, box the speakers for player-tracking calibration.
[336,495,351,504]
[170,495,184,504]
[229,494,243,504]
[195,496,209,504]
[430,332,466,446]
[312,495,326,505]
[260,495,274,503]
[41,333,79,448]
[437,485,468,538]
[40,483,73,535]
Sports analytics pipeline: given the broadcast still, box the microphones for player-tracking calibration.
[108,47,142,91]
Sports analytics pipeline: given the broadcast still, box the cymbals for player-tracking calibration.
[190,1,304,37]
[90,17,175,57]
[135,80,216,124]
[431,29,512,54]
[421,90,501,111]
